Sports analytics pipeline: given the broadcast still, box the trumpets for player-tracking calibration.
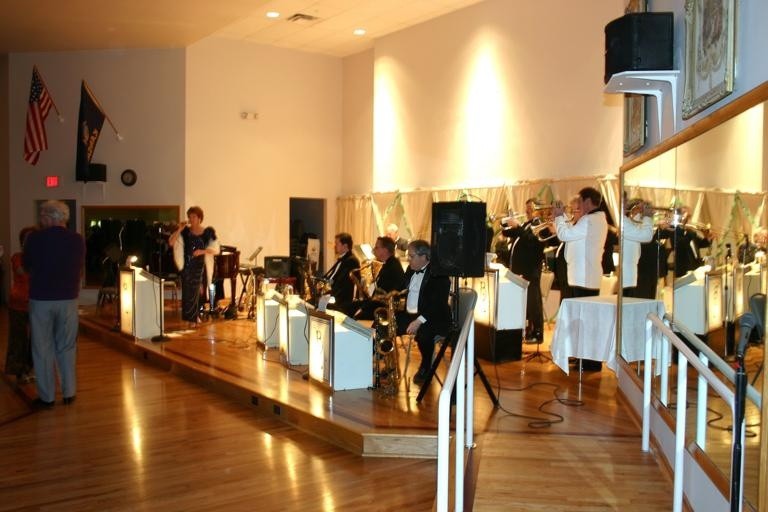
[530,209,581,242]
[531,201,572,219]
[627,204,711,246]
[489,209,528,231]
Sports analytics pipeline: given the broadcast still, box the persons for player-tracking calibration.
[355,237,405,353]
[395,239,451,382]
[168,207,220,328]
[623,195,715,300]
[501,186,618,372]
[321,232,361,318]
[21,199,86,410]
[6,227,38,385]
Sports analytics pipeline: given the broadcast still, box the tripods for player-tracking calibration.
[416,277,499,409]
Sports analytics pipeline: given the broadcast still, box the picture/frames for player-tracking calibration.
[678,0,738,121]
[618,0,650,160]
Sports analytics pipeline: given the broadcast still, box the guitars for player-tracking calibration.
[303,270,319,305]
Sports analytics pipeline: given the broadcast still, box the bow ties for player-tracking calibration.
[416,270,423,274]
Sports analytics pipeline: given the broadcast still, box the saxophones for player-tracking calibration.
[349,262,408,381]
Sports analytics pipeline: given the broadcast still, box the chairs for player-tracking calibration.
[259,254,297,287]
[414,284,479,389]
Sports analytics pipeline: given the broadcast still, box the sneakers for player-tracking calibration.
[33,398,55,407]
[64,397,75,404]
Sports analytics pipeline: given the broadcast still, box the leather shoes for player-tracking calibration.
[414,366,428,382]
[524,336,543,344]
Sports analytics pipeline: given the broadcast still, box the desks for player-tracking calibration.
[540,267,553,298]
[546,290,622,405]
[600,274,619,295]
[623,292,673,387]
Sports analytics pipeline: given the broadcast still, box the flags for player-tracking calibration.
[23,66,53,166]
[76,82,105,184]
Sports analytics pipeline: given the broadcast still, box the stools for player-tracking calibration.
[92,285,121,323]
[162,279,181,315]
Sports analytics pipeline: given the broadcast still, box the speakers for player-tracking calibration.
[604,11,674,84]
[431,201,486,278]
[264,256,295,277]
[80,163,106,182]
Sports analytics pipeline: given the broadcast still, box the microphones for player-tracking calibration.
[734,313,756,360]
[176,220,192,226]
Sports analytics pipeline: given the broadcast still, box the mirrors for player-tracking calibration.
[79,201,183,292]
[34,197,79,232]
[616,82,768,511]
